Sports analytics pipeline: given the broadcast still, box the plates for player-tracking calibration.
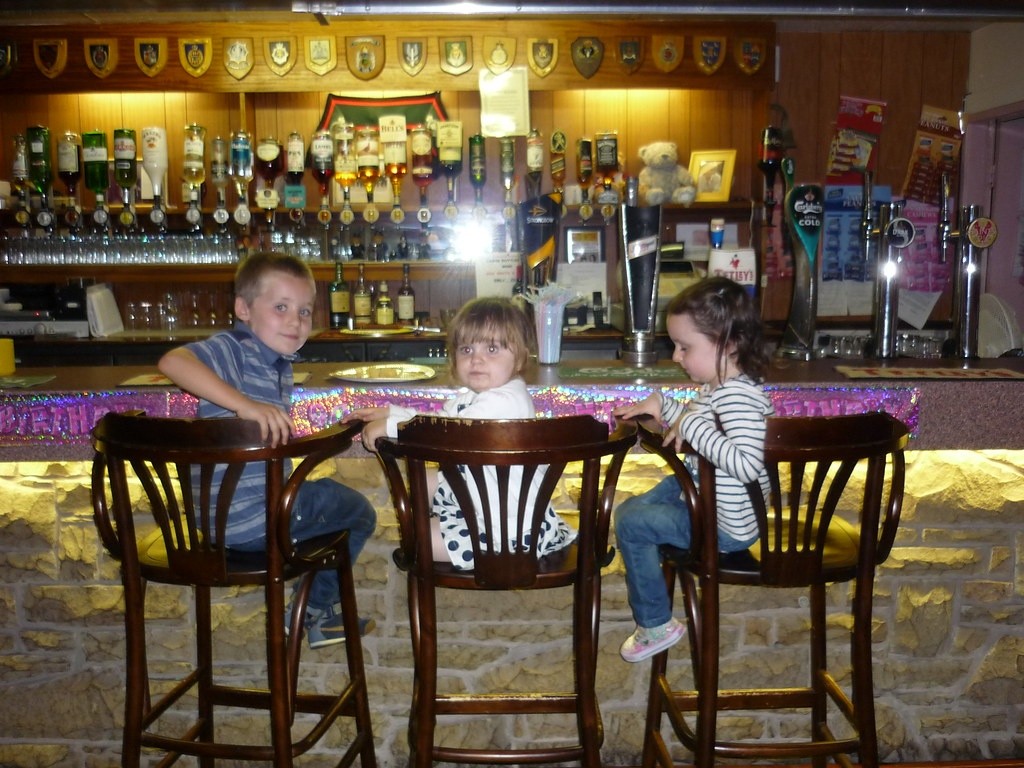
[329,362,438,386]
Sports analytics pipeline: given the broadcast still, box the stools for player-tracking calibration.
[373,414,639,768]
[643,408,907,768]
[86,408,369,768]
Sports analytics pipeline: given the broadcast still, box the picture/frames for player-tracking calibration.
[563,225,606,264]
[525,37,559,79]
[133,37,168,79]
[691,35,728,77]
[262,35,298,77]
[480,34,519,75]
[650,34,685,72]
[0,39,19,80]
[570,36,605,80]
[83,38,119,79]
[688,149,738,202]
[304,34,338,77]
[32,38,68,79]
[344,35,386,81]
[396,37,428,76]
[222,36,256,80]
[177,36,214,78]
[437,35,474,76]
[614,35,646,75]
[732,35,769,76]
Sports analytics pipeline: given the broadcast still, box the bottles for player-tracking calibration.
[373,280,394,325]
[330,225,429,262]
[353,263,373,325]
[10,131,620,217]
[328,261,350,328]
[710,216,725,251]
[397,262,415,326]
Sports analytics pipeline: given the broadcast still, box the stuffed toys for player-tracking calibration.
[638,141,698,205]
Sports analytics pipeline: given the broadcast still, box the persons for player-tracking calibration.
[157,251,377,649]
[339,296,578,572]
[612,276,776,660]
[700,161,722,192]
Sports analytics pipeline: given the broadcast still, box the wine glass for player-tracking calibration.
[122,285,239,335]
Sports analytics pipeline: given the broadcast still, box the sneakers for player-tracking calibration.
[308,612,376,649]
[285,604,315,641]
[620,617,687,663]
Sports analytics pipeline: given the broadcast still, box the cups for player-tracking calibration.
[440,308,456,332]
[0,338,16,379]
[813,333,943,359]
[0,227,322,263]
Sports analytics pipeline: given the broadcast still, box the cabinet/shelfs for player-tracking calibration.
[0,203,517,361]
